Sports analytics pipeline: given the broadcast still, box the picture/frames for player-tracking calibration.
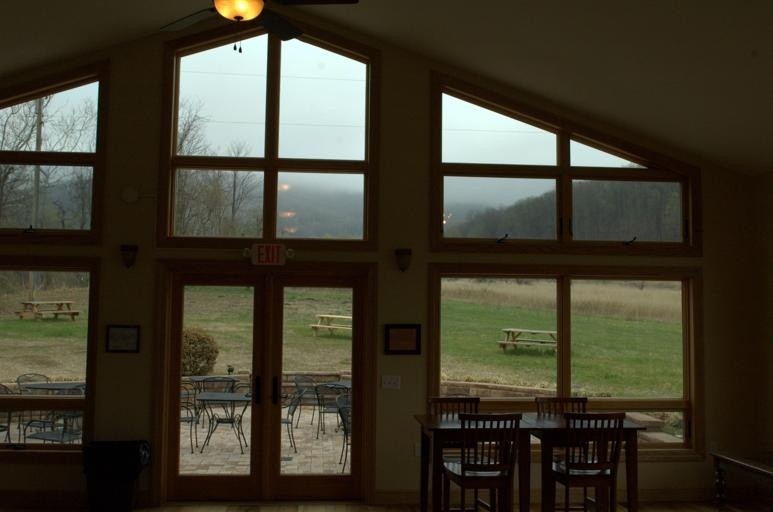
[105,324,141,353]
[385,324,421,355]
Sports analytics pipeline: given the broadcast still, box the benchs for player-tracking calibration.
[309,324,352,337]
[510,336,557,343]
[37,311,79,322]
[14,310,33,320]
[496,341,557,355]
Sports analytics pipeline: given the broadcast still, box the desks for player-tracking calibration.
[413,413,647,512]
[501,329,557,355]
[19,300,75,320]
[312,314,352,337]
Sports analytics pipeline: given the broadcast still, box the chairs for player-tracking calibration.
[182,370,251,455]
[1,374,86,443]
[428,396,625,512]
[280,374,351,473]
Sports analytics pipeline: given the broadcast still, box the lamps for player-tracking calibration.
[213,0,264,52]
